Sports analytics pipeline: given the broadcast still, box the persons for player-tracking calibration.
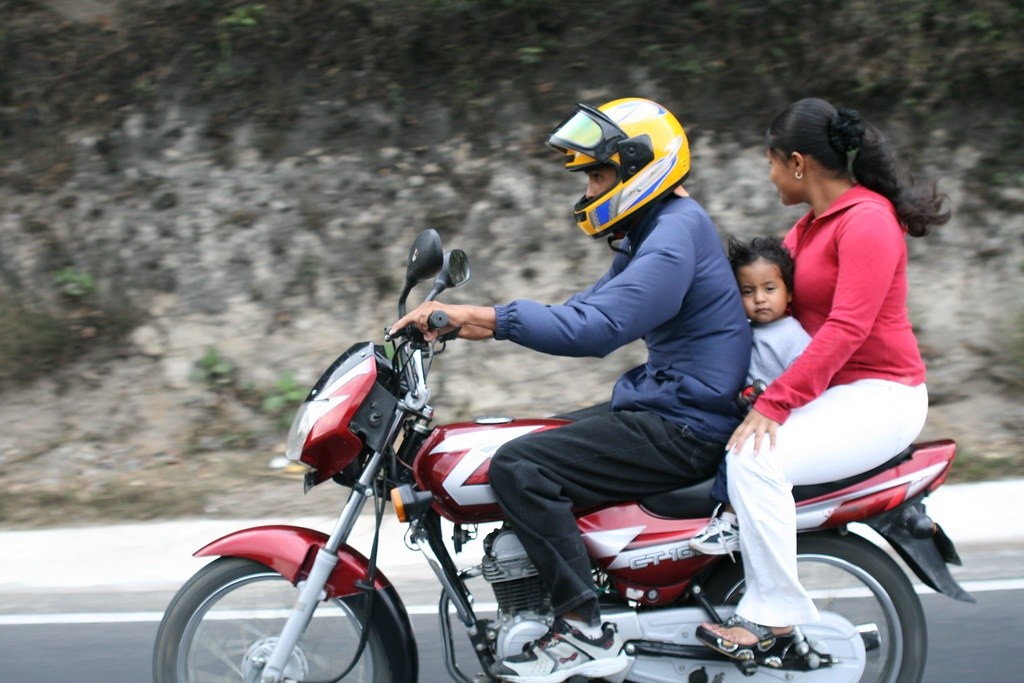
[391,97,753,683]
[690,237,813,555]
[696,98,952,662]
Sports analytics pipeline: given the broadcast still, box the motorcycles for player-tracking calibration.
[150,229,976,683]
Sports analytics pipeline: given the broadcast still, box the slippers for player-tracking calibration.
[696,614,796,661]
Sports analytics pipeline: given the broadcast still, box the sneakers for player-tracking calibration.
[490,618,628,683]
[688,503,741,563]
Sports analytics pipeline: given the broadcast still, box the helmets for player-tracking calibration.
[546,97,690,239]
